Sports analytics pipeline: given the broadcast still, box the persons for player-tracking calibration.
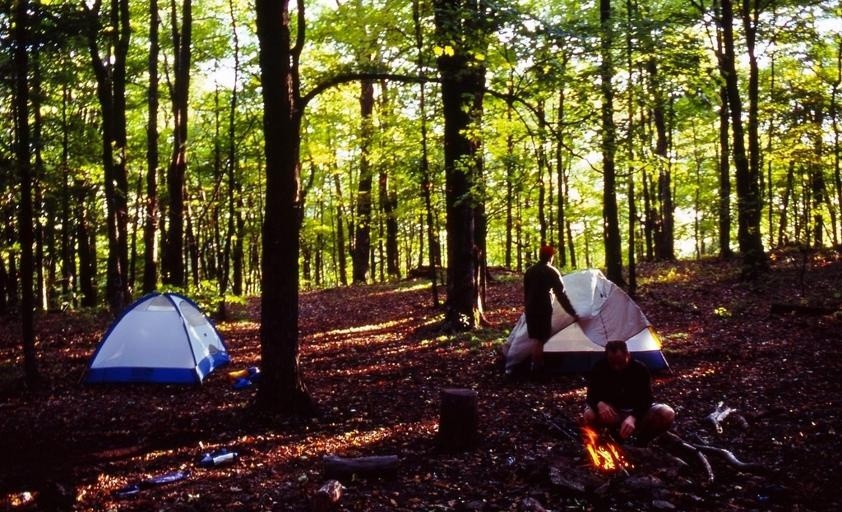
[524,247,578,373]
[582,340,675,444]
[80,291,229,386]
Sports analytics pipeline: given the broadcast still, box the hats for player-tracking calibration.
[540,245,558,254]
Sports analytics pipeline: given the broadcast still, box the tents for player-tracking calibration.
[502,268,673,377]
